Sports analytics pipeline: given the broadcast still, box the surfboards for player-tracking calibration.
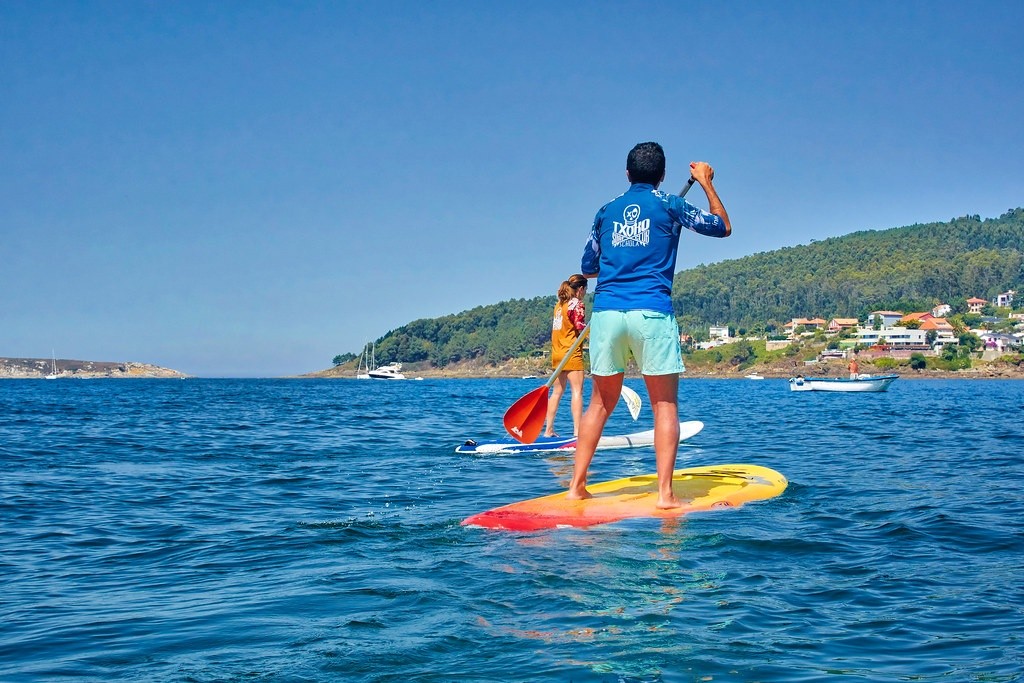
[456,419,705,458]
[460,463,788,532]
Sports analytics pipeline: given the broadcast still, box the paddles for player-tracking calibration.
[501,161,697,444]
[582,332,641,419]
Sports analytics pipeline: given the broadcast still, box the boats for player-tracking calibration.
[788,375,900,392]
[368,366,407,380]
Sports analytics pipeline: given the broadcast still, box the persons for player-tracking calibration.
[543,273,588,437]
[570,139,730,511]
[847,357,858,379]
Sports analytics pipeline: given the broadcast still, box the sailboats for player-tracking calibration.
[357,341,375,379]
